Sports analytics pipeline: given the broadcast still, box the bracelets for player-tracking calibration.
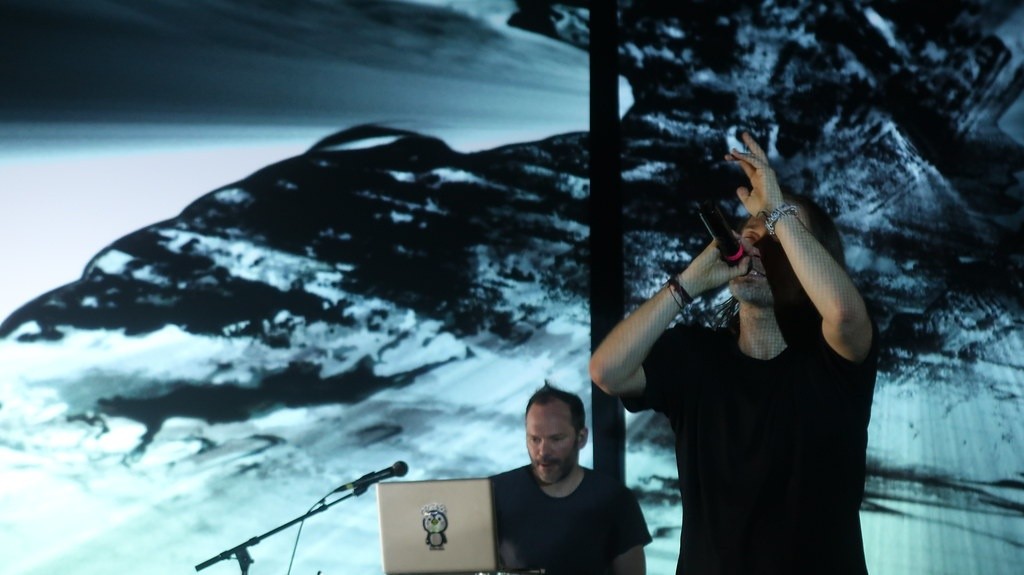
[766,205,795,234]
[666,276,693,308]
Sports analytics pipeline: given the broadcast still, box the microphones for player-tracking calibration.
[334,461,408,493]
[698,201,753,276]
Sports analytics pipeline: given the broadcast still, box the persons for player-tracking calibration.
[490,384,653,575]
[589,131,877,575]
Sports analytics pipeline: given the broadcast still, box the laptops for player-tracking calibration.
[376,478,546,575]
